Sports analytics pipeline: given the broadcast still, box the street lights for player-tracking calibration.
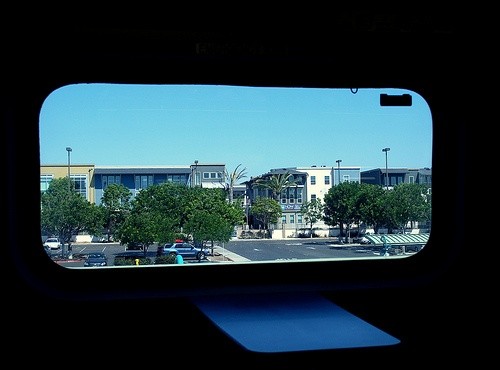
[88,169,94,204]
[66,147,72,251]
[336,160,343,237]
[382,149,390,190]
[195,161,198,188]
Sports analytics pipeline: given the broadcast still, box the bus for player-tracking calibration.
[124,244,146,259]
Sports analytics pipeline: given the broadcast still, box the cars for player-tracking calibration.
[43,238,60,250]
[83,253,107,267]
[353,238,370,245]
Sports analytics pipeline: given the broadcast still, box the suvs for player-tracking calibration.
[162,243,211,262]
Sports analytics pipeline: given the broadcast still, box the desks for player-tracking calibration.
[186,288,402,370]
